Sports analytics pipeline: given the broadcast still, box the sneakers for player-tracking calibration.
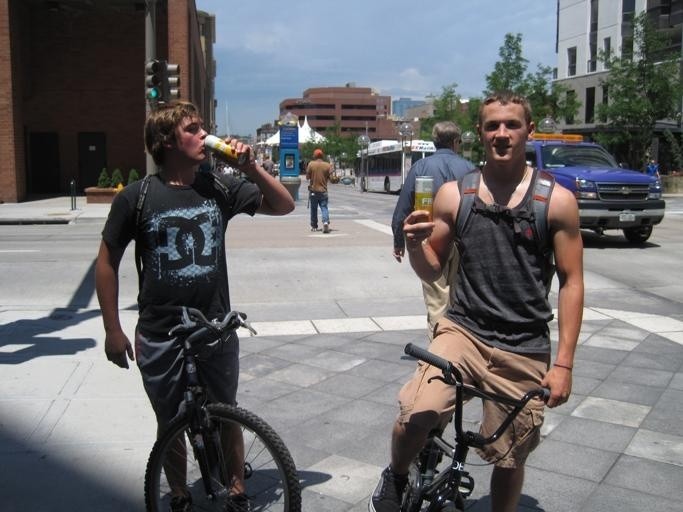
[311,221,330,233]
[224,490,254,512]
[368,463,410,512]
[169,491,193,512]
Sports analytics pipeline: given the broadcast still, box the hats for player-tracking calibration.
[313,149,323,157]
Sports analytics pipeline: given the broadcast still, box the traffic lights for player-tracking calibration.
[147,60,163,104]
[165,65,181,101]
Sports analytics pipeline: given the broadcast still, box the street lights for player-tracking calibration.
[341,152,348,176]
[399,123,413,191]
[357,135,370,191]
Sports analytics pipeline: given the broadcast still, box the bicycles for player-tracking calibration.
[144,311,303,512]
[387,342,561,512]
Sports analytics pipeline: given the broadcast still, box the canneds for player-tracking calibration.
[414,176,434,233]
[204,134,244,164]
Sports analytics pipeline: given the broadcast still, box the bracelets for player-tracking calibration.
[553,363,572,370]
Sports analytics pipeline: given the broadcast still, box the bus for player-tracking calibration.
[356,139,436,196]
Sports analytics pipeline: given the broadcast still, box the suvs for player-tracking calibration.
[527,132,666,244]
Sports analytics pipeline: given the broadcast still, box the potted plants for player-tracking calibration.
[85,163,145,204]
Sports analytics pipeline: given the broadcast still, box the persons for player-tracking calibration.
[372,90,586,512]
[260,153,273,175]
[217,164,242,178]
[388,120,478,343]
[304,150,341,233]
[93,99,295,512]
[647,157,660,177]
